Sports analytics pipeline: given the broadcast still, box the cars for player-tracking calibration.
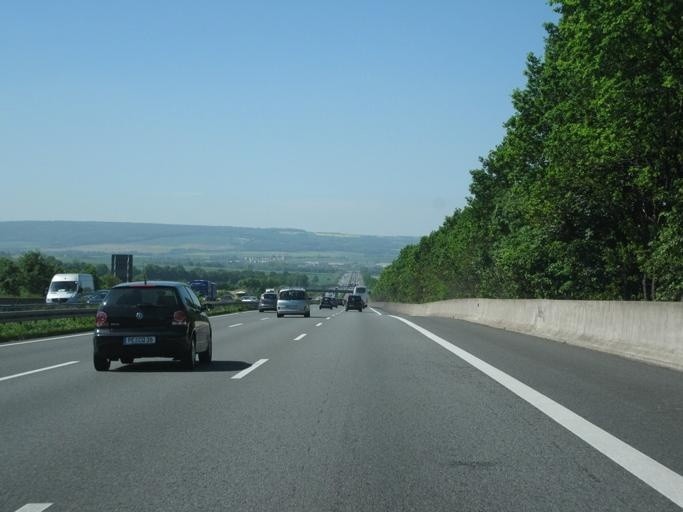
[259,293,276,313]
[85,290,107,306]
[276,288,312,319]
[318,296,345,309]
[218,291,258,302]
[92,279,214,374]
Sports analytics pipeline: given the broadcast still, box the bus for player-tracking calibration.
[353,286,368,308]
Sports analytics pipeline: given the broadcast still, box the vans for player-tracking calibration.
[46,273,94,307]
[346,296,363,312]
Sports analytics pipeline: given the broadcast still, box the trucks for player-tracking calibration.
[192,280,217,301]
